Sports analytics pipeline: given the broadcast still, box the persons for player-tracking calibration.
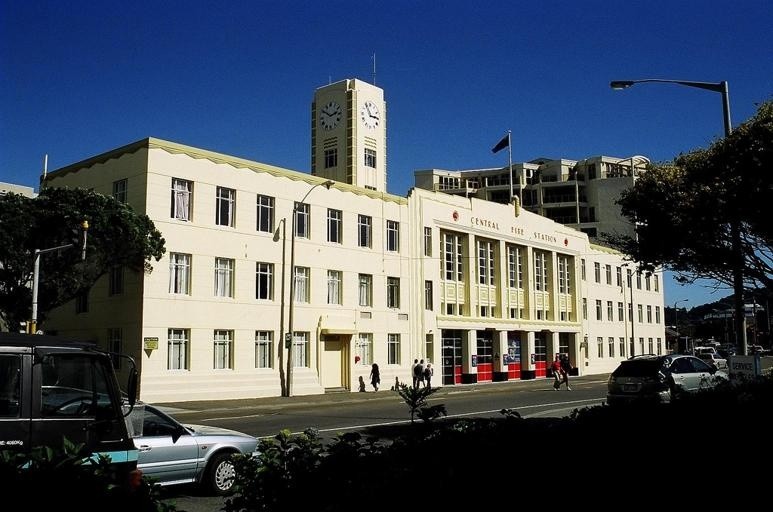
[412,359,433,388]
[370,364,380,391]
[551,353,573,391]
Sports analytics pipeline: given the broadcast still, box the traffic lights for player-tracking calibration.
[69,228,83,256]
[19,321,31,334]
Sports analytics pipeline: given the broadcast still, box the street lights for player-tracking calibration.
[674,298,688,332]
[285,180,336,396]
[610,78,746,353]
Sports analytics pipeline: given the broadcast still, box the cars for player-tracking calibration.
[693,339,770,368]
[44,394,264,497]
[606,354,729,404]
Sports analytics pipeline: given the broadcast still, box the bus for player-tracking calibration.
[0,329,140,487]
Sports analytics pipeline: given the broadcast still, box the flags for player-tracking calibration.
[492,134,509,153]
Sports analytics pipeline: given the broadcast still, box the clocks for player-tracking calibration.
[319,100,341,131]
[362,100,381,130]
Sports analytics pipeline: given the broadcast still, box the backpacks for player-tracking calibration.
[425,369,429,376]
[414,364,423,377]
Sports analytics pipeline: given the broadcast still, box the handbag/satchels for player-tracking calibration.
[378,379,380,384]
